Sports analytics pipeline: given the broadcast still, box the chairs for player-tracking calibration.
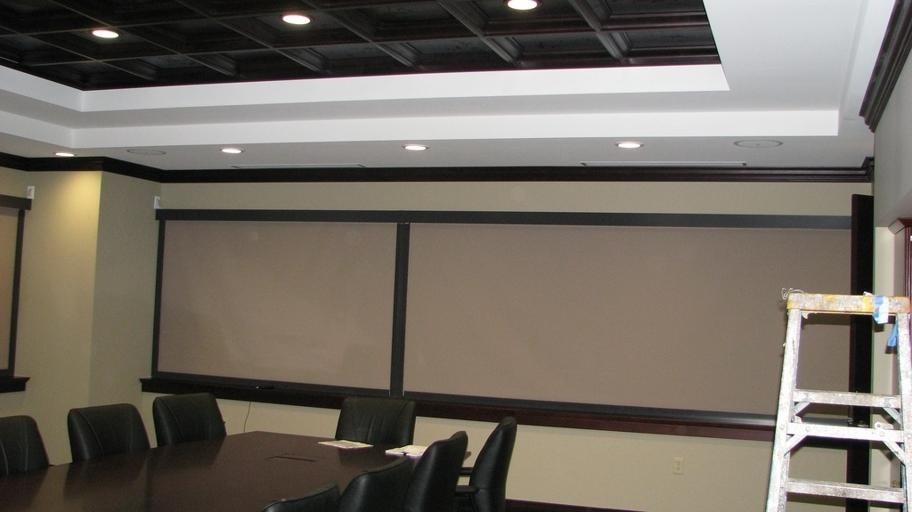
[67,402,151,465]
[152,393,228,447]
[404,431,468,512]
[452,415,516,512]
[259,483,341,512]
[338,456,414,512]
[336,396,418,453]
[0,415,57,480]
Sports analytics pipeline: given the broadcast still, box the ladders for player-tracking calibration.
[765,293,912,512]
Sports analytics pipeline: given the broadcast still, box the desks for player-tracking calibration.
[0,430,472,512]
